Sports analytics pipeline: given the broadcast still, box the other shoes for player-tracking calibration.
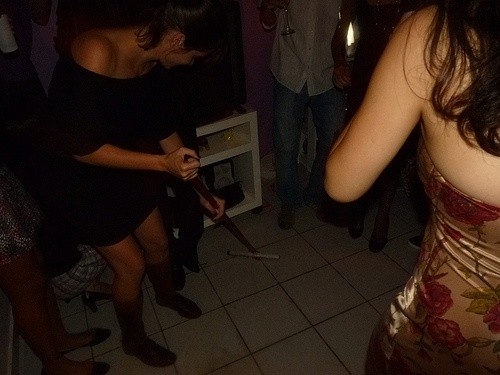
[369,217,391,252]
[168,226,201,273]
[169,260,185,290]
[61,327,112,356]
[39,360,112,375]
[347,214,366,238]
[277,202,297,229]
[408,235,424,250]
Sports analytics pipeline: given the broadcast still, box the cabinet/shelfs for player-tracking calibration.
[165,105,262,238]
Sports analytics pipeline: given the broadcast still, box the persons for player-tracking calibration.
[0,0,230,375]
[254,0,431,254]
[325,0,500,375]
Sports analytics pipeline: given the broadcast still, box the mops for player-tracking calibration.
[189,176,282,259]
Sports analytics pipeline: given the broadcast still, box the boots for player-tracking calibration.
[112,302,178,369]
[144,262,202,319]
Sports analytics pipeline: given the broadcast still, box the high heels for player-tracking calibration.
[81,290,109,311]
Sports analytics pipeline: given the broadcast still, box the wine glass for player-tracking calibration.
[280,0,295,35]
[341,45,356,111]
